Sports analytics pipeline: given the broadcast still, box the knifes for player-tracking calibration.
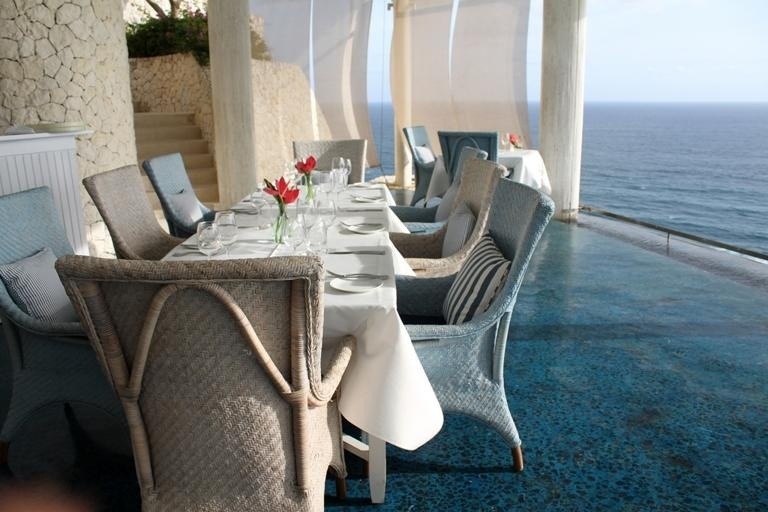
[329,250,385,255]
[347,208,383,212]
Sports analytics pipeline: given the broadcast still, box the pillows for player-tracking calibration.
[0,245,75,324]
[442,200,476,257]
[439,232,510,325]
[165,188,204,231]
[423,160,458,222]
[414,145,436,165]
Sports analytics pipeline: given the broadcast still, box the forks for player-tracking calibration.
[324,267,391,281]
[168,247,230,257]
[337,218,383,227]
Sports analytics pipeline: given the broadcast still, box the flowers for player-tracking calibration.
[261,176,300,242]
[509,135,518,147]
[293,155,316,199]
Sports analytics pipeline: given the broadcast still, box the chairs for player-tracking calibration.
[0,188,135,477]
[402,124,445,207]
[54,253,356,511]
[437,129,499,185]
[142,152,218,237]
[392,177,557,475]
[387,158,506,277]
[292,139,367,185]
[80,161,189,262]
[386,144,488,234]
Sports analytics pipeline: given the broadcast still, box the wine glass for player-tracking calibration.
[283,157,353,256]
[196,158,302,258]
[500,133,510,154]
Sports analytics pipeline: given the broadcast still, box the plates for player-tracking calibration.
[346,223,385,235]
[355,195,384,203]
[329,272,383,294]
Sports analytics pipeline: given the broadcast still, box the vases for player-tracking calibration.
[301,177,316,204]
[275,213,291,245]
